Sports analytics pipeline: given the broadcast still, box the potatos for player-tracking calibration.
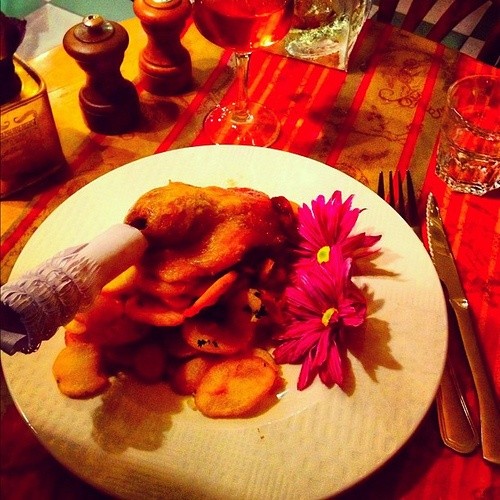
[52,261,284,419]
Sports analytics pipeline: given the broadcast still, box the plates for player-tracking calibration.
[1,145,447,500]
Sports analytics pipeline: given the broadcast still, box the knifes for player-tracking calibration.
[427,192,499,463]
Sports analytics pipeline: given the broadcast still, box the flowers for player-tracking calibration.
[287,191,383,273]
[269,258,371,389]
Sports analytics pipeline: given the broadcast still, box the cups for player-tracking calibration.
[434,76,500,197]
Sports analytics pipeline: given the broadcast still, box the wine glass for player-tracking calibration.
[192,0,294,148]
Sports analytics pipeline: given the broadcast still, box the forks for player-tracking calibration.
[377,170,479,455]
